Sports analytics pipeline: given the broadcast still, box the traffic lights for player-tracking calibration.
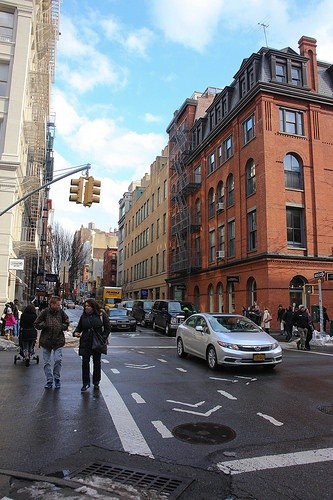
[70,177,82,203]
[84,177,101,207]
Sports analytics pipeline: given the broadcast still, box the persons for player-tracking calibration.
[241,303,272,335]
[224,317,232,330]
[20,305,37,367]
[277,304,330,351]
[73,299,111,391]
[184,304,201,321]
[34,296,70,390]
[2,299,19,340]
[33,298,49,312]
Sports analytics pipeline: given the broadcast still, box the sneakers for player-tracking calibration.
[55,381,61,389]
[94,385,99,390]
[81,384,90,391]
[44,382,52,389]
[296,342,310,351]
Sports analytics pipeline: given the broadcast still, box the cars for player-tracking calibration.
[67,302,74,308]
[106,307,136,332]
[176,312,283,371]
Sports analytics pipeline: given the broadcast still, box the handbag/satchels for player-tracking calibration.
[91,328,108,355]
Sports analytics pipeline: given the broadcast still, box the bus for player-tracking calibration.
[95,286,122,310]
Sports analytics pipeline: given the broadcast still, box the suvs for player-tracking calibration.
[123,301,134,315]
[149,299,198,336]
[132,300,155,327]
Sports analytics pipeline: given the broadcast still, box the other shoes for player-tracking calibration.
[25,356,29,367]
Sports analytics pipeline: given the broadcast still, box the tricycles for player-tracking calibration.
[14,330,39,367]
